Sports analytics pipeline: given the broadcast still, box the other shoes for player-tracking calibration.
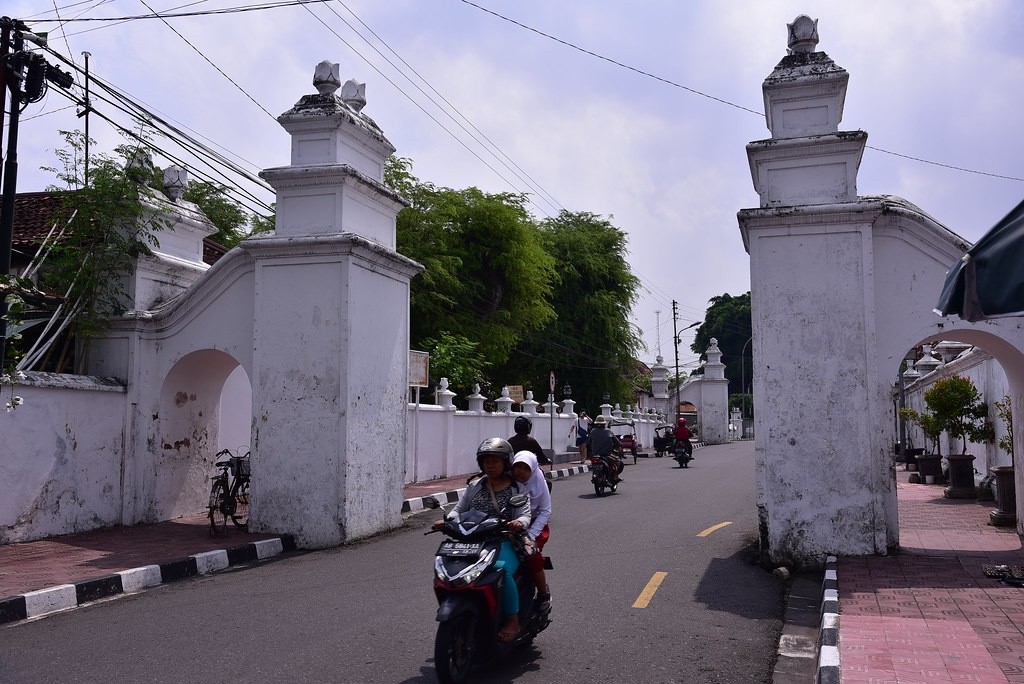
[614,477,622,483]
[581,460,585,463]
[536,590,552,615]
[689,457,694,460]
[673,457,678,460]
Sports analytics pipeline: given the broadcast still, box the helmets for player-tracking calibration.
[678,418,686,425]
[477,437,514,473]
[514,416,532,435]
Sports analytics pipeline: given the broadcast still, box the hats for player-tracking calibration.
[594,415,607,424]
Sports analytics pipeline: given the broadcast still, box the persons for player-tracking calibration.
[587,415,624,480]
[433,438,552,643]
[568,409,593,464]
[673,418,694,460]
[507,416,552,494]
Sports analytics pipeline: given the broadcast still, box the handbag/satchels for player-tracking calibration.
[577,427,588,438]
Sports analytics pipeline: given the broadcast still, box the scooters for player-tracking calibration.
[423,492,554,684]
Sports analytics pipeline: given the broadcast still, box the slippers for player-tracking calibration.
[496,625,522,642]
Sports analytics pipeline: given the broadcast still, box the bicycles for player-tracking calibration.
[205,444,251,540]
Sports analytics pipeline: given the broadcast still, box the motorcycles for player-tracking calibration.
[586,444,626,497]
[672,436,695,468]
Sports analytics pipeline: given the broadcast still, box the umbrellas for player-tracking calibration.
[933,199,1024,322]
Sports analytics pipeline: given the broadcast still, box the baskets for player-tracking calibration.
[230,456,250,476]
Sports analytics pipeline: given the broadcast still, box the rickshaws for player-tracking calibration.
[653,422,676,457]
[607,419,638,464]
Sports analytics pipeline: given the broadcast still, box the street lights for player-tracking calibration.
[674,321,702,427]
